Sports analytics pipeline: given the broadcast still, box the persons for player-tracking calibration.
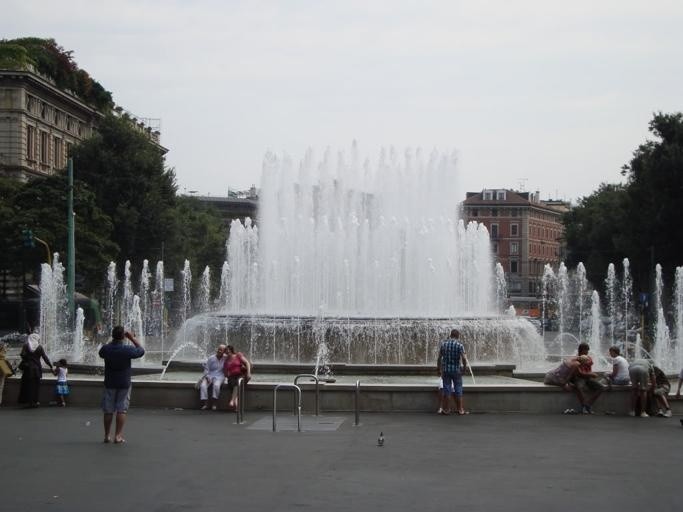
[602,346,631,385]
[221,345,251,413]
[648,365,672,418]
[97,324,146,444]
[16,333,54,407]
[198,343,227,410]
[626,358,653,417]
[0,344,7,406]
[435,328,471,416]
[570,342,603,414]
[543,354,593,391]
[49,359,69,407]
[675,368,683,426]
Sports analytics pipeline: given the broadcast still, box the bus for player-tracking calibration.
[502,295,559,319]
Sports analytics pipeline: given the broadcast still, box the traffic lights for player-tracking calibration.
[22,227,35,249]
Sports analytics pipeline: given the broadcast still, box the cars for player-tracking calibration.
[93,307,169,340]
[522,310,651,346]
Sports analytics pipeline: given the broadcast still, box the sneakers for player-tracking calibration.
[563,409,569,415]
[664,410,672,419]
[570,408,577,415]
[629,410,636,417]
[656,409,663,418]
[586,405,595,414]
[456,409,470,416]
[641,411,649,418]
[581,404,589,415]
[437,408,451,416]
